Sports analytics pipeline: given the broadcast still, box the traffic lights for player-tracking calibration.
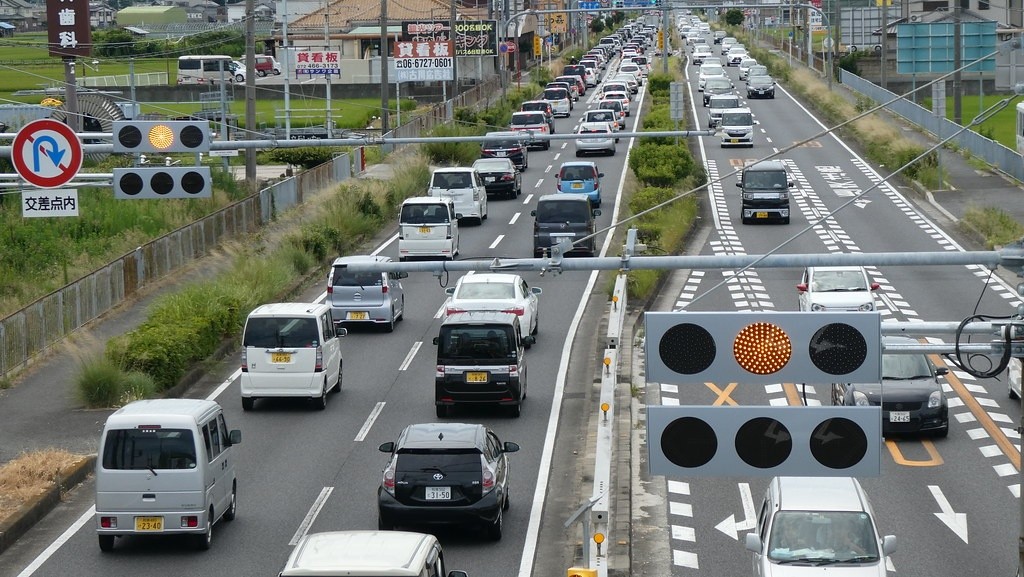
[112,167,212,198]
[644,308,882,385]
[110,120,210,155]
[642,403,877,477]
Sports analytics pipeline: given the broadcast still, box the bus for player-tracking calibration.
[176,54,236,84]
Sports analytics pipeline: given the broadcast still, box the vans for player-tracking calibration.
[676,8,776,149]
[737,163,794,224]
[428,13,662,225]
[744,475,898,577]
[531,194,602,258]
[396,197,459,261]
[324,255,405,334]
[433,315,527,416]
[277,530,473,577]
[239,301,348,410]
[93,397,242,553]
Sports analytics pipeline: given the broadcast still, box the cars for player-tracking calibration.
[378,420,524,543]
[796,265,880,314]
[1006,355,1023,400]
[830,333,950,436]
[446,272,541,347]
[230,56,284,83]
